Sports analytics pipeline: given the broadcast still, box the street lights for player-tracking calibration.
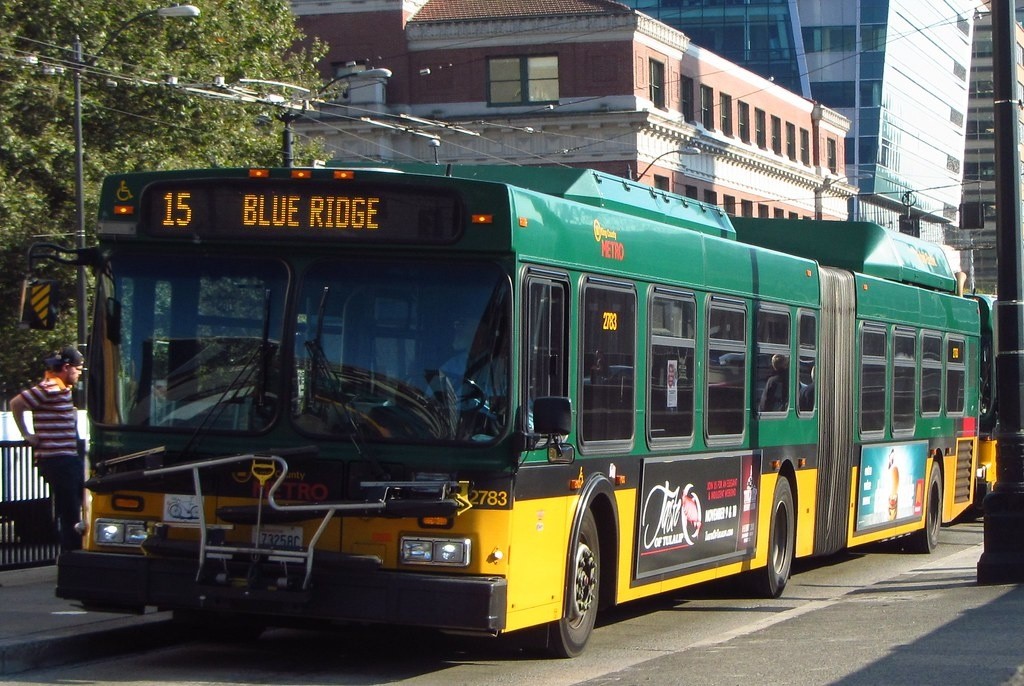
[813,173,873,220]
[68,5,200,411]
[277,66,392,168]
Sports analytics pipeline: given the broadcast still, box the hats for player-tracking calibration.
[44,346,84,366]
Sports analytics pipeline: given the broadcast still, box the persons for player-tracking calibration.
[10,346,90,601]
[758,351,802,413]
[423,300,515,442]
[589,348,612,385]
[799,364,818,412]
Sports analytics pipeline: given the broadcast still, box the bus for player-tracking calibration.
[18,163,979,662]
[965,289,1001,524]
